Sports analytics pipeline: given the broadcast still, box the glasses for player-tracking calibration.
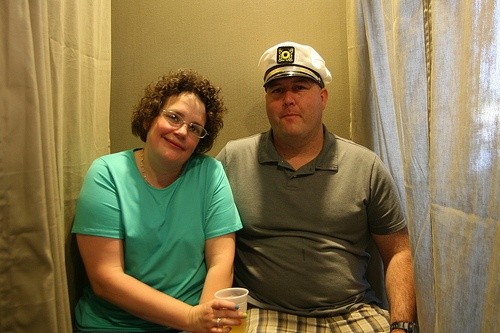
[160,109,209,138]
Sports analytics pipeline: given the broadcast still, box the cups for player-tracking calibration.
[214,288,249,333]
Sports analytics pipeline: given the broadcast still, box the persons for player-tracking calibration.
[71,68,248,333]
[215,41,418,333]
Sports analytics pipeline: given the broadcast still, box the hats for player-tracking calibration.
[258,41,332,89]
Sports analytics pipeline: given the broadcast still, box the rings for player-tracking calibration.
[217,318,221,327]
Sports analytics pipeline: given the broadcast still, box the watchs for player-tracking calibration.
[388,321,419,333]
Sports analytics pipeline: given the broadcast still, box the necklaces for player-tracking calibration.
[140,149,151,185]
